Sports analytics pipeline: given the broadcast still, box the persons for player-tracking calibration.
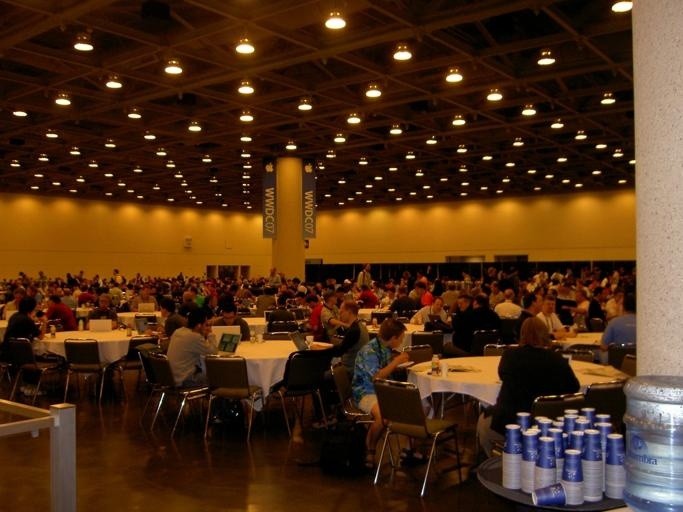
[1,267,282,338]
[349,319,428,471]
[477,316,580,458]
[312,299,371,430]
[164,307,226,425]
[270,261,636,351]
[0,296,49,397]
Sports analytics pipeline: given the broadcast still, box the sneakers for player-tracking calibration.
[24,388,43,396]
[312,415,336,428]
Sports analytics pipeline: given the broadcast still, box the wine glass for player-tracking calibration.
[305,335,313,350]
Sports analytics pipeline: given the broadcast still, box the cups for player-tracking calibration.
[249,328,263,343]
[440,362,448,377]
[501,407,626,507]
[372,318,377,330]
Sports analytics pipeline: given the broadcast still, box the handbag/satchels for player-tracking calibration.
[320,421,367,479]
[220,398,245,440]
[252,407,296,438]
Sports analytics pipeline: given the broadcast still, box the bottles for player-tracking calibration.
[431,354,439,376]
[49,325,55,336]
[126,322,132,336]
[77,319,83,332]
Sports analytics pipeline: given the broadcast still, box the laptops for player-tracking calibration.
[135,318,158,336]
[89,319,112,332]
[216,333,243,356]
[289,330,311,350]
[139,303,155,312]
[262,331,289,340]
[427,313,440,324]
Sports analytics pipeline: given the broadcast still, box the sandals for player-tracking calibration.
[399,447,430,463]
[368,448,376,471]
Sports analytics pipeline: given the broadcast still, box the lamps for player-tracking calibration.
[5,0,636,180]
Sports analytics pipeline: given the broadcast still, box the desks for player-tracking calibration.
[478,454,632,512]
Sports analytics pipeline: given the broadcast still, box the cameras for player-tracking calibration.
[35,310,42,317]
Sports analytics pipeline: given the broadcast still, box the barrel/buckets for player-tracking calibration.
[620,374,683,512]
